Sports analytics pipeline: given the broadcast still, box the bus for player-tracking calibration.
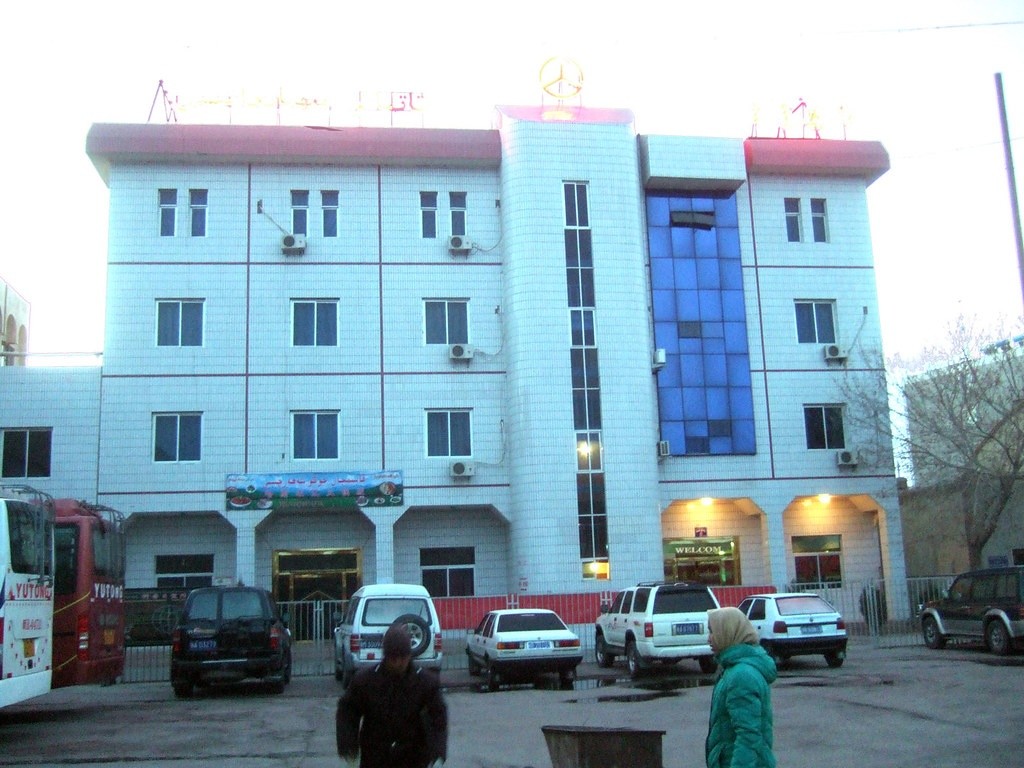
[30,497,126,690]
[0,484,56,708]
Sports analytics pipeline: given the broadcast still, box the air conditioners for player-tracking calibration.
[823,344,848,361]
[449,459,475,476]
[448,235,473,251]
[656,440,670,458]
[836,450,858,466]
[280,233,306,253]
[652,348,666,368]
[450,343,474,359]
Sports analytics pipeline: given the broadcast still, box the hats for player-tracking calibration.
[383,623,410,656]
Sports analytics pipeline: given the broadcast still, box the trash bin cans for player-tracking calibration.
[541,724,667,768]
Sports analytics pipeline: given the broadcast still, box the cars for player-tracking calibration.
[465,608,584,686]
[736,593,848,666]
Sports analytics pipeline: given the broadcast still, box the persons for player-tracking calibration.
[336,624,448,768]
[705,606,777,768]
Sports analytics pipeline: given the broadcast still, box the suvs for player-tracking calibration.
[170,586,292,697]
[331,583,442,687]
[917,569,1024,655]
[594,581,723,678]
